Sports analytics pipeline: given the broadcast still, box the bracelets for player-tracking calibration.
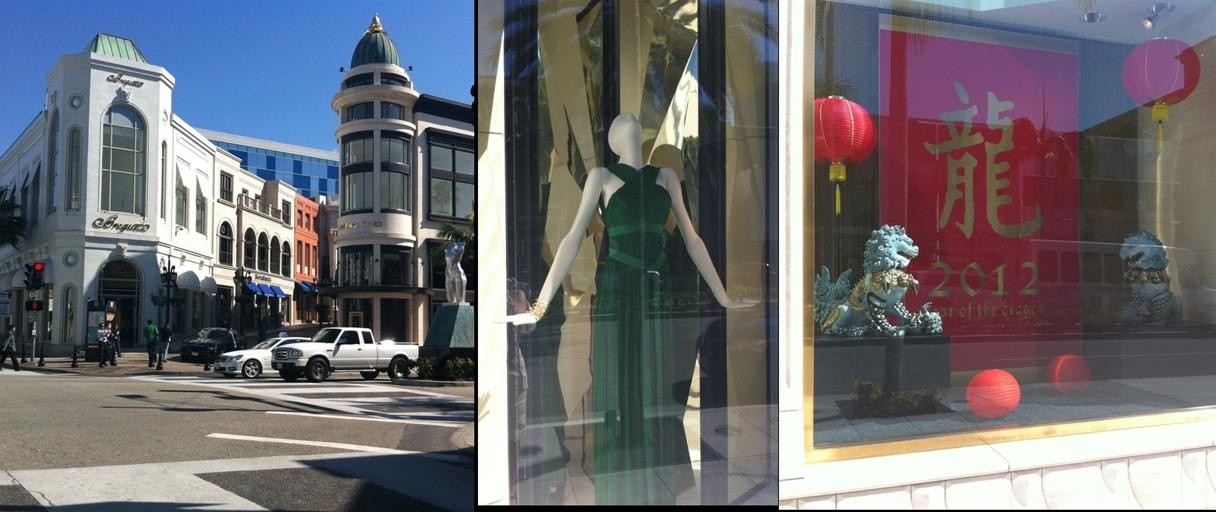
[526,298,552,322]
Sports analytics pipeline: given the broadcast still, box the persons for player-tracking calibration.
[505,111,763,505]
[96,323,116,368]
[159,322,173,361]
[115,328,122,357]
[102,322,115,366]
[443,240,469,303]
[143,319,159,367]
[1,323,23,371]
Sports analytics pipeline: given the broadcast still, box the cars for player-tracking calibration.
[213,336,309,378]
[180,328,246,364]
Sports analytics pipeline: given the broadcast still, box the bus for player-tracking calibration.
[1021,238,1215,334]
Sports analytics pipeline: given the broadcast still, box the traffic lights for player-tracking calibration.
[26,300,44,311]
[23,262,34,289]
[33,260,44,273]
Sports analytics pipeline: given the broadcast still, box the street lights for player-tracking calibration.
[311,278,335,327]
[234,267,254,332]
[159,265,178,328]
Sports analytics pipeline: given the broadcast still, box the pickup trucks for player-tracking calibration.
[272,326,421,381]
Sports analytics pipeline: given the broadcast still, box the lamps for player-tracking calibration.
[1143,3,1177,32]
